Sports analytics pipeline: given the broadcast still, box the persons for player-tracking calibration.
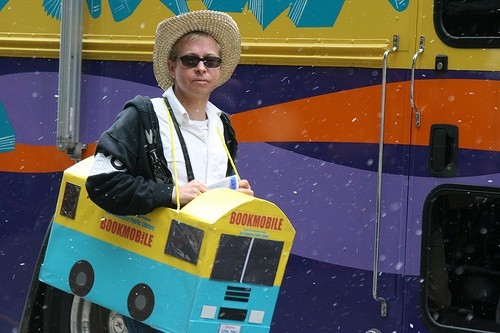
[86,10,254,333]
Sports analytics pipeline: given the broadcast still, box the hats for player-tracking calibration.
[152,10,242,93]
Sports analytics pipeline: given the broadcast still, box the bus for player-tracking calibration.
[0,0,500,333]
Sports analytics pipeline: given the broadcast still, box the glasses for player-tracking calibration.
[174,55,222,68]
